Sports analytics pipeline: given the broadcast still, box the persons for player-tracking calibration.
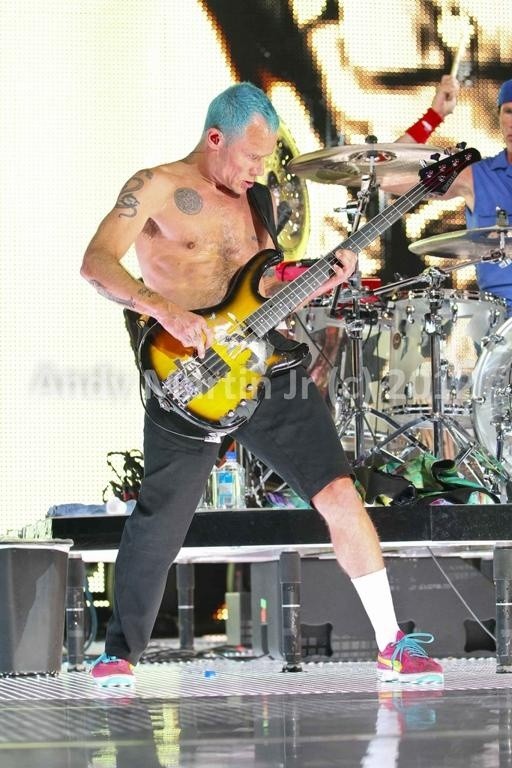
[94,674,403,768]
[77,80,450,689]
[366,73,512,344]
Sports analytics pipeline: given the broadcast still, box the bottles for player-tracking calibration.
[216,451,246,511]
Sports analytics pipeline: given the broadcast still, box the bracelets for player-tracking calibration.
[406,104,444,145]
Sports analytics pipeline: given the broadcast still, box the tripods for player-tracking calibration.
[361,254,512,504]
[335,154,430,509]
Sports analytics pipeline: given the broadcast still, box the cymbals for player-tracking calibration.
[288,142,450,188]
[409,225,512,261]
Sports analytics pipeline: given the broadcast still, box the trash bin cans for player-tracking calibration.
[0,538,75,676]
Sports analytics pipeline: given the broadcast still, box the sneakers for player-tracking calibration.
[89,653,137,687]
[375,630,444,684]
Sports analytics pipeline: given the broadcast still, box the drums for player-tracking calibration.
[287,295,389,442]
[388,291,508,429]
[471,315,512,478]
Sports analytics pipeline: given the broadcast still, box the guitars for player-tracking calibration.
[137,142,482,435]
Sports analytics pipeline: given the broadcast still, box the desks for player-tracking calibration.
[52,503,512,673]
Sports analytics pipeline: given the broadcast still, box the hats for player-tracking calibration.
[498,79,512,111]
